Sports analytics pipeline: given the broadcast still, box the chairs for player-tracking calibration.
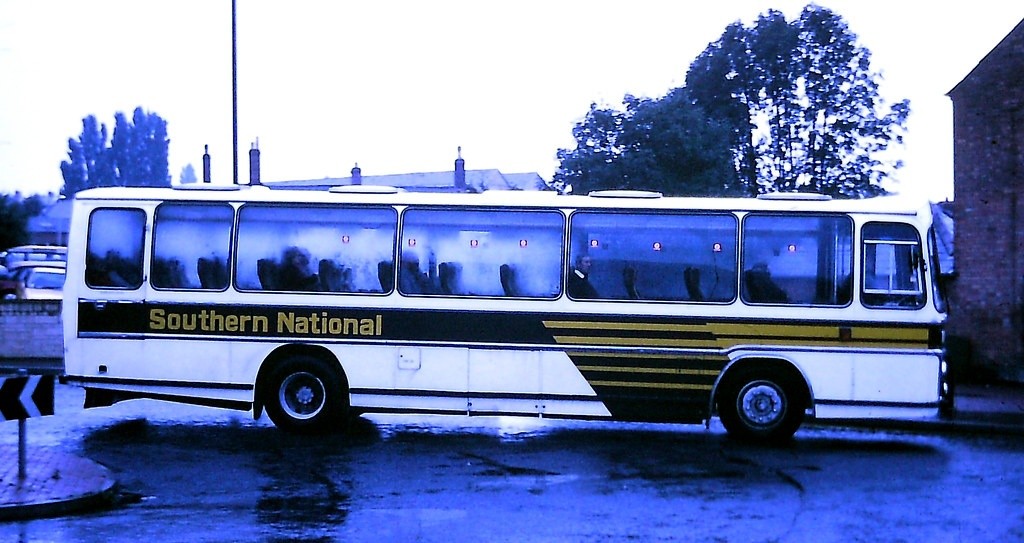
[90,252,796,308]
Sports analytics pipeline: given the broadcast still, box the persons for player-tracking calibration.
[103,249,136,288]
[277,246,320,291]
[837,272,874,306]
[747,260,789,303]
[400,250,439,295]
[568,253,600,299]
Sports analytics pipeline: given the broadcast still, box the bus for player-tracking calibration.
[58,182,953,441]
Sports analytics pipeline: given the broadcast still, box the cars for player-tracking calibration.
[0,244,69,279]
[15,265,66,300]
